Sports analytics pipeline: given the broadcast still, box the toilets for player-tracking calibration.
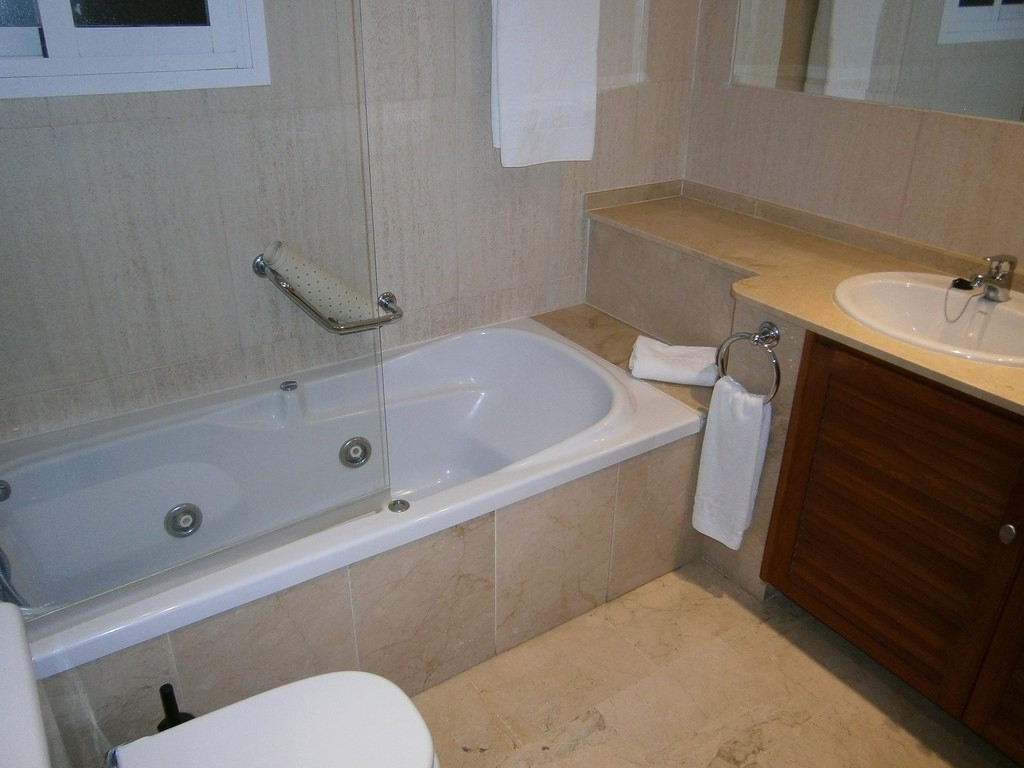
[0,599,442,766]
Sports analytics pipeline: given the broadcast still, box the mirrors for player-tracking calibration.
[734,0,1024,127]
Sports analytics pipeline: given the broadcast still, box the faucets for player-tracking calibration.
[968,254,1020,303]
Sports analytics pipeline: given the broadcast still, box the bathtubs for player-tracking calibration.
[0,318,708,768]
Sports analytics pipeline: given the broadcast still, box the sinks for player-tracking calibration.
[833,270,1023,368]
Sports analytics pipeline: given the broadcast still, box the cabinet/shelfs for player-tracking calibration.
[759,326,1024,761]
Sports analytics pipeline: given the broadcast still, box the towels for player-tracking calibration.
[629,333,723,387]
[690,374,771,552]
[803,1,886,101]
[490,0,601,169]
